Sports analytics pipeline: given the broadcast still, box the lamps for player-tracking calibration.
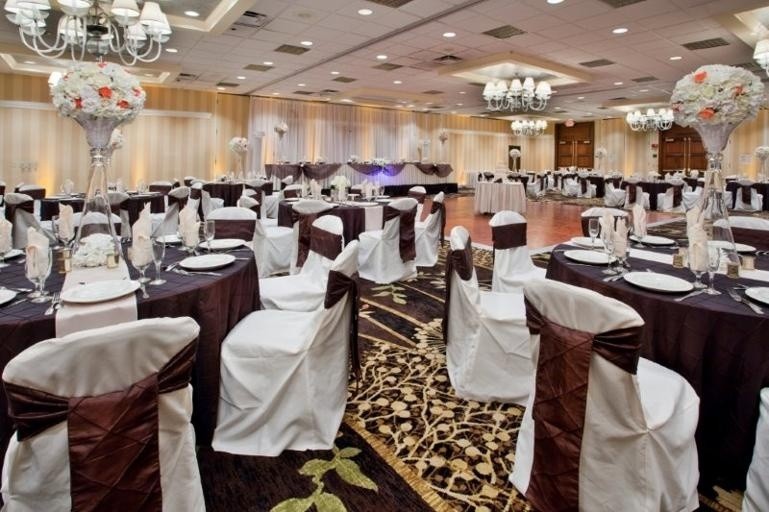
[5,0,173,70]
[482,73,556,112]
[511,119,547,138]
[624,106,675,133]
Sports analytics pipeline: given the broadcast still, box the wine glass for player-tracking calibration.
[300,182,385,201]
[23,216,216,304]
[589,218,721,295]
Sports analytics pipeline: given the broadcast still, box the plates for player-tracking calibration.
[0,235,245,305]
[563,234,769,305]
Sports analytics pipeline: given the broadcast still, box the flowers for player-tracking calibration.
[671,61,767,164]
[230,136,246,152]
[45,58,148,154]
[273,122,288,138]
[507,147,523,158]
[594,146,608,160]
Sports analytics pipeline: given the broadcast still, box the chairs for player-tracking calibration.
[508,276,702,512]
[438,223,530,408]
[0,315,207,512]
[210,237,361,456]
